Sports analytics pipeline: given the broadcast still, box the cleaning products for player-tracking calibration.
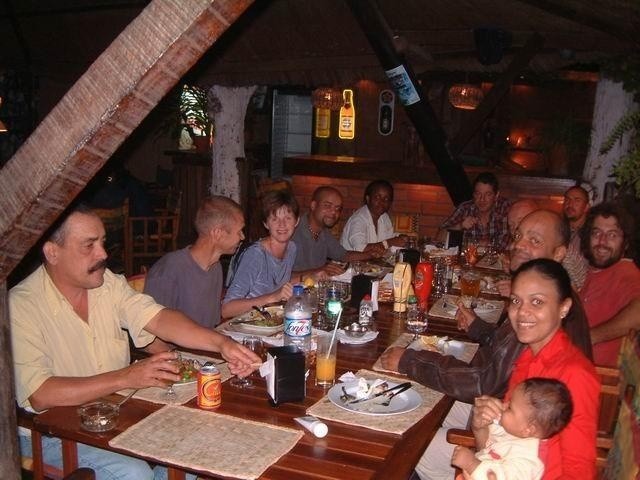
[413,262,434,312]
[393,263,411,312]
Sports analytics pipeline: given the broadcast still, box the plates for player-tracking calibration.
[174,356,211,387]
[328,381,423,415]
[239,308,285,333]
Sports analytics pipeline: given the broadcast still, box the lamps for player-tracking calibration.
[309,86,344,112]
[447,83,481,110]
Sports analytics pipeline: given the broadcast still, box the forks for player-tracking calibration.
[204,360,227,366]
[252,306,271,320]
[380,385,413,407]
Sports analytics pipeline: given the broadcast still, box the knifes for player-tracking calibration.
[348,382,410,404]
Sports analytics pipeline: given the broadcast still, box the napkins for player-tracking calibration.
[466,249,506,272]
[335,259,397,287]
[372,325,480,384]
[451,269,512,298]
[304,365,447,438]
[113,349,240,408]
[427,291,506,328]
[216,307,291,340]
[107,402,304,480]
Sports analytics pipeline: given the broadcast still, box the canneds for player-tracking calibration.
[327,288,341,318]
[197,366,222,409]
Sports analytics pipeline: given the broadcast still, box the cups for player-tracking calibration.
[243,336,265,359]
[442,260,454,291]
[462,272,481,296]
[316,335,337,388]
[350,260,360,276]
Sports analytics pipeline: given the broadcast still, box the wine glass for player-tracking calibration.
[163,352,180,401]
[406,306,428,342]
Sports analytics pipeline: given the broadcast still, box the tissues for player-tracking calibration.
[446,229,463,253]
[398,249,420,270]
[259,345,306,405]
[351,276,380,313]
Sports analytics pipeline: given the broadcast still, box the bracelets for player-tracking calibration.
[227,375,255,389]
[299,273,304,282]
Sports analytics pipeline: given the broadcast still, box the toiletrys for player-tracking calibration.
[293,415,328,437]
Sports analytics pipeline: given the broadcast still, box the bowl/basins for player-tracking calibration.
[344,324,368,338]
[80,401,119,433]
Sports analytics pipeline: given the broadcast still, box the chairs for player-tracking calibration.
[0,268,190,480]
[443,326,640,479]
[16,174,230,325]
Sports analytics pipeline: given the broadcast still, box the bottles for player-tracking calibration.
[282,275,345,380]
[407,295,417,312]
[315,90,355,139]
[360,294,372,326]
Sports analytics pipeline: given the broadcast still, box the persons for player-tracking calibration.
[341,177,411,251]
[6,206,261,480]
[382,172,640,480]
[143,194,245,338]
[220,188,326,321]
[294,183,378,277]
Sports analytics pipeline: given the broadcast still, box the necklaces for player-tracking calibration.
[305,221,322,240]
